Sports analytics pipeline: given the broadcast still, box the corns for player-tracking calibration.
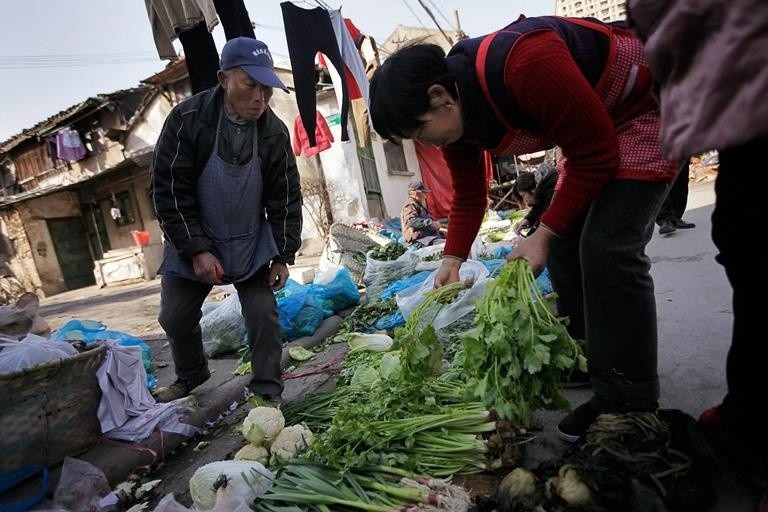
[209,472,232,512]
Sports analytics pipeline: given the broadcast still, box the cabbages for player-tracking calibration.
[343,331,394,356]
[377,349,409,383]
[350,363,380,392]
[187,458,274,509]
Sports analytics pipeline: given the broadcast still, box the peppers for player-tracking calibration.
[371,242,408,262]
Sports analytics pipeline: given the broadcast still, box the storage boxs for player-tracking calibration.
[0,340,107,486]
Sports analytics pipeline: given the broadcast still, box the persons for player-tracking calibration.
[623,0,768,450]
[151,37,303,408]
[513,164,564,237]
[656,157,694,236]
[400,179,445,251]
[366,13,685,442]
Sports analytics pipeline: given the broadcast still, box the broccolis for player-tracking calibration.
[237,393,285,443]
[271,419,318,465]
[233,441,273,469]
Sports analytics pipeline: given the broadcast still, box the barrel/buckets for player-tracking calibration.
[129,229,150,247]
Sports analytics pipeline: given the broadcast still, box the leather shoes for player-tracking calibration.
[659,219,695,234]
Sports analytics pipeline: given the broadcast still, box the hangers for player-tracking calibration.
[280,0,330,12]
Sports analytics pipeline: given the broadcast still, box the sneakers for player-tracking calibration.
[159,371,211,402]
[557,396,660,443]
[258,394,284,408]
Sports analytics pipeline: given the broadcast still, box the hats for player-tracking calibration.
[408,180,431,193]
[221,36,291,94]
